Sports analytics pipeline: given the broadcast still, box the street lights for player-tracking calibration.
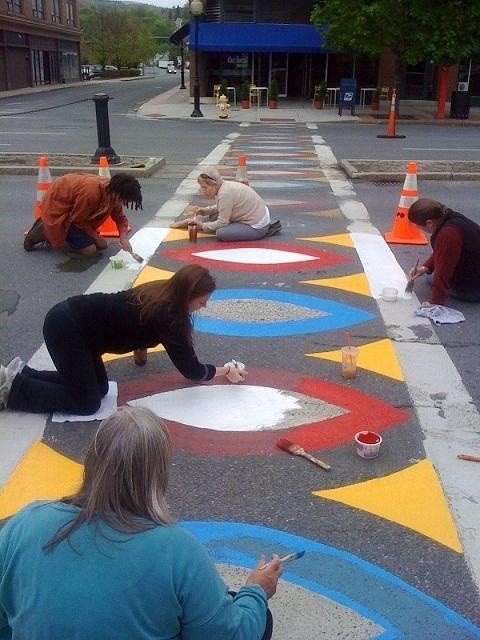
[175,17,187,89]
[190,1,203,117]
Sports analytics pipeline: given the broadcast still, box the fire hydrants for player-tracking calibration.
[217,94,230,119]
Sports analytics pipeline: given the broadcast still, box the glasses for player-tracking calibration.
[200,173,216,182]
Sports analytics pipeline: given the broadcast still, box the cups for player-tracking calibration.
[340,346,359,379]
[188,220,197,243]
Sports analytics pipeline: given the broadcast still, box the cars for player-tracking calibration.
[167,66,176,74]
[104,66,117,71]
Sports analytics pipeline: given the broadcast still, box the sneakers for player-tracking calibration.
[24,217,48,250]
[0,357,26,408]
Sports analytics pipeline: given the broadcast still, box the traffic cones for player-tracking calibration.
[385,163,428,245]
[96,156,131,238]
[377,88,406,138]
[237,155,250,185]
[24,158,52,236]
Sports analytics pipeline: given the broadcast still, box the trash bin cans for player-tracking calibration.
[338,78,357,116]
[448,81,472,119]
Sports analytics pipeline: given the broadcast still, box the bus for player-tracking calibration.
[159,61,173,69]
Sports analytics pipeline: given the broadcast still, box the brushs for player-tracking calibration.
[119,242,143,263]
[259,550,305,570]
[405,257,420,294]
[277,438,331,471]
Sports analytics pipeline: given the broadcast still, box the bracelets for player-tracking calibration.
[226,366,230,374]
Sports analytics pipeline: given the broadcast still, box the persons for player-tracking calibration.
[407,199,480,307]
[188,166,283,243]
[1,401,284,640]
[0,263,248,416]
[24,172,144,255]
